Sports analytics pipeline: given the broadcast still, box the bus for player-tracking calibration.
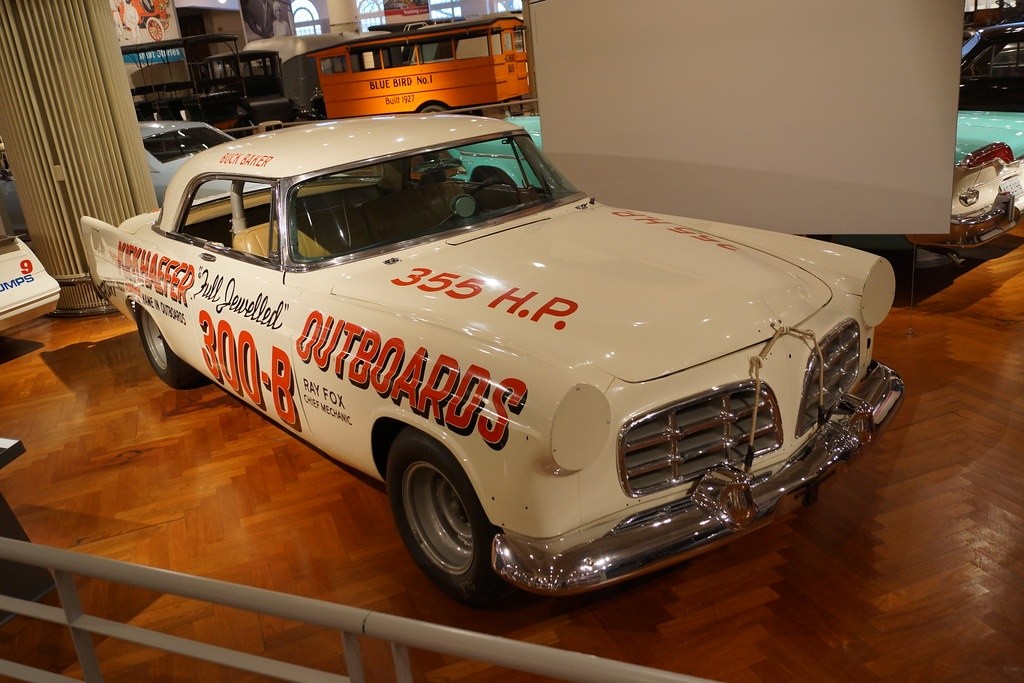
[308,17,528,119]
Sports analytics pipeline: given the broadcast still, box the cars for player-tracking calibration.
[0,122,260,238]
[118,34,301,131]
[909,22,1023,250]
[80,109,904,595]
[1,215,60,339]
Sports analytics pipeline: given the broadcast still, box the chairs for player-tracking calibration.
[356,179,465,243]
[231,204,370,258]
